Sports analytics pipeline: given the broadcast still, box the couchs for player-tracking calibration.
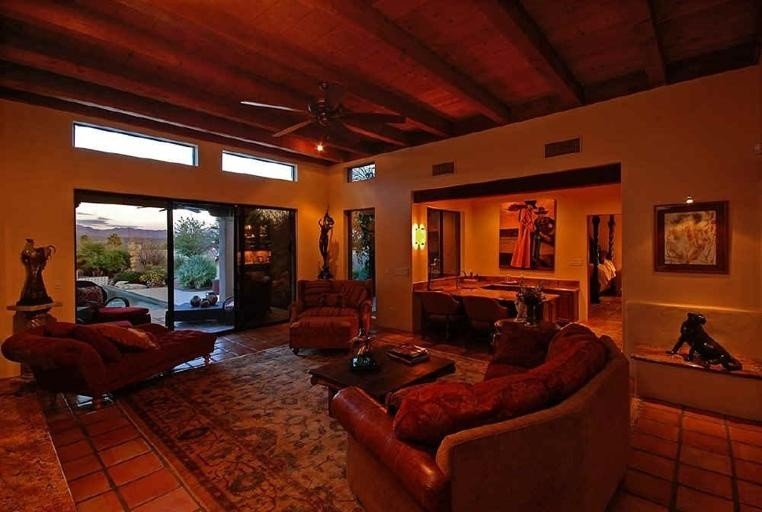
[0,318,217,404]
[331,317,632,510]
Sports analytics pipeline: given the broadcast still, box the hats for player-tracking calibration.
[525,200,538,209]
[534,207,548,215]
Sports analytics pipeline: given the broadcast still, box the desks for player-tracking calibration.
[9,301,63,382]
[631,348,761,424]
[167,299,231,325]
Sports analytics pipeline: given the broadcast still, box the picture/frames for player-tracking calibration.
[653,199,729,273]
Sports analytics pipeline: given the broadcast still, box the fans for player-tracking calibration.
[239,82,407,148]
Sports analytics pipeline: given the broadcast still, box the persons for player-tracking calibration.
[530,207,553,269]
[316,203,336,271]
[509,201,538,271]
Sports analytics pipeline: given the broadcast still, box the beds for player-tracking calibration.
[588,214,618,304]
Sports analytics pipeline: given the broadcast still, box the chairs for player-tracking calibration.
[288,277,374,352]
[415,274,579,351]
[77,276,155,327]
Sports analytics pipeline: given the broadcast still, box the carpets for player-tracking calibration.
[115,341,647,512]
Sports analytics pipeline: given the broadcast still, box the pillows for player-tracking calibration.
[43,321,151,363]
